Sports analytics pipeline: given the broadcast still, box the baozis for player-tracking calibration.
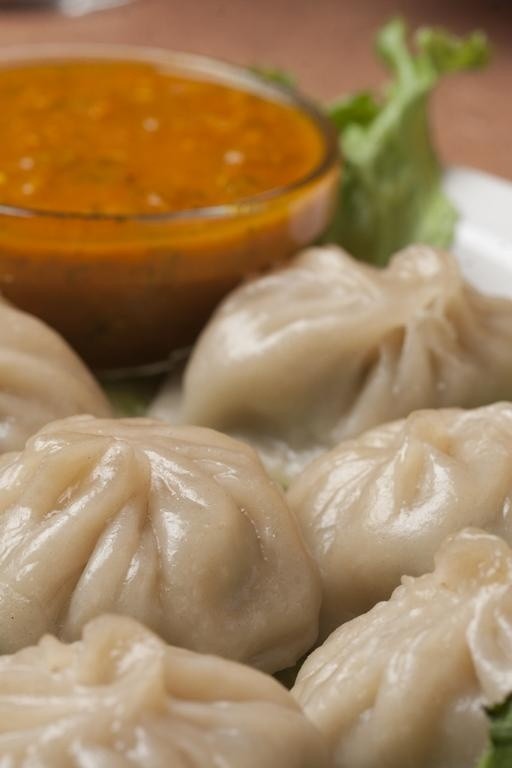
[1,243,512,766]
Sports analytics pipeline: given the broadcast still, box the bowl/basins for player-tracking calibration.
[3,45,350,383]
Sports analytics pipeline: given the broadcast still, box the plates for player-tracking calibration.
[446,160,512,299]
